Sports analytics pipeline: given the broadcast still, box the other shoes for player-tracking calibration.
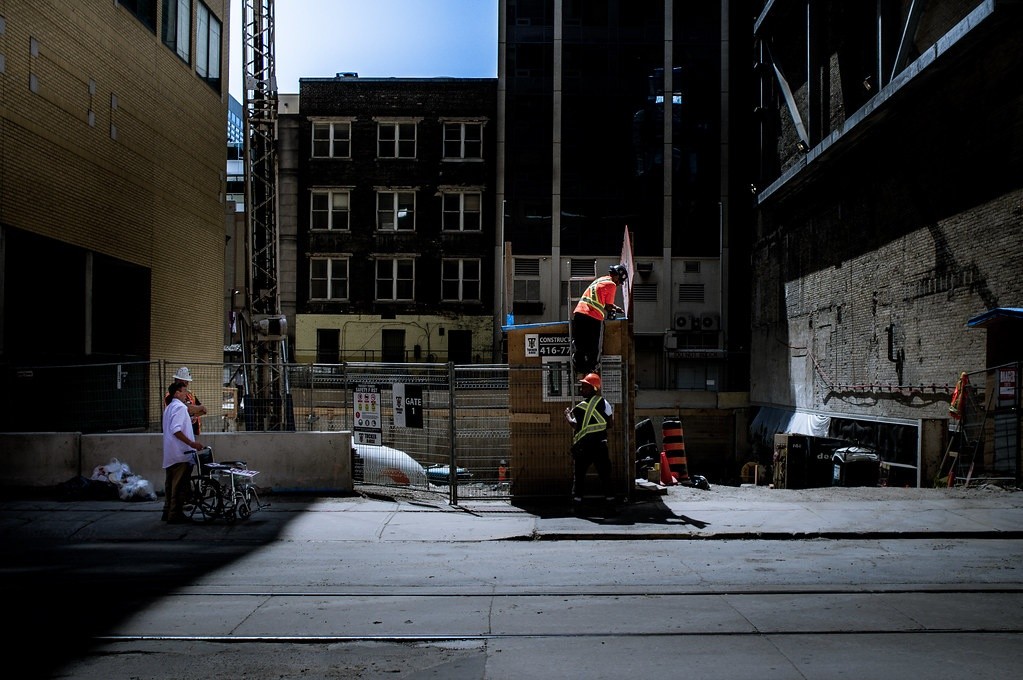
[162,518,193,524]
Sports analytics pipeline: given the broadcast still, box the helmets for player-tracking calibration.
[609,265,628,284]
[500,460,506,464]
[579,373,601,389]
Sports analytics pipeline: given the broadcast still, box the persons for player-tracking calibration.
[564,373,619,514]
[572,265,628,381]
[161,367,207,522]
[495,460,510,491]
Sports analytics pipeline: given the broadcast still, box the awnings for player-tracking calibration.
[968,307,1023,329]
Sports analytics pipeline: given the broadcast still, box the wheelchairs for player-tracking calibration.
[177,445,272,526]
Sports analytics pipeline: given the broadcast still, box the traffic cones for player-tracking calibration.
[659,452,679,486]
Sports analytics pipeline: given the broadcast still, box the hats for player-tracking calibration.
[172,367,192,382]
